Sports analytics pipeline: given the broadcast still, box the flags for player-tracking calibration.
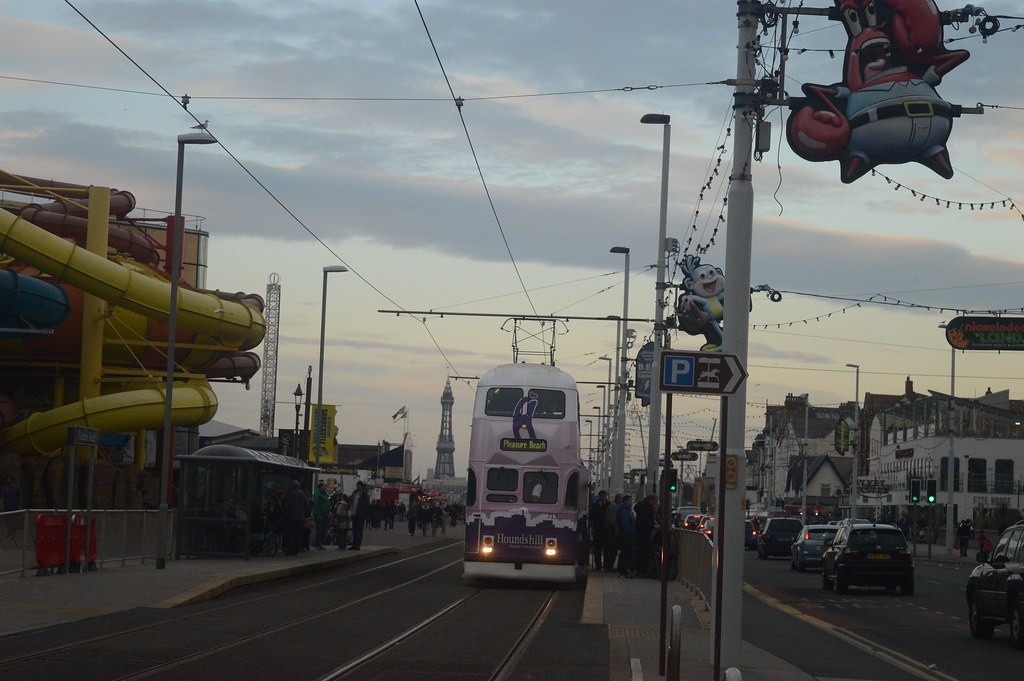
[308,405,335,464]
[392,405,407,423]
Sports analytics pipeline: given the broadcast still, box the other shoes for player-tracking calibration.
[336,545,360,551]
[315,543,326,550]
[284,552,297,557]
[593,567,617,572]
[408,531,415,536]
[960,553,967,557]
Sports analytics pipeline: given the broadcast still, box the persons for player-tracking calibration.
[671,513,683,528]
[956,518,975,557]
[890,516,909,541]
[138,471,154,510]
[999,520,1010,536]
[879,511,885,524]
[820,552,836,592]
[589,490,658,579]
[366,499,464,538]
[0,475,23,550]
[215,480,367,557]
[527,476,547,503]
[977,530,993,558]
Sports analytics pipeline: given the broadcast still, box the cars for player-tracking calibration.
[745,518,872,573]
[675,506,714,543]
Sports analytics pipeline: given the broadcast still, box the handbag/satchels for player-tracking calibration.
[953,541,960,549]
[304,517,314,529]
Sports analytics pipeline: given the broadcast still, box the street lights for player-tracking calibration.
[846,364,859,519]
[313,266,348,494]
[800,393,809,526]
[641,113,671,499]
[606,315,620,500]
[155,132,217,570]
[610,246,630,494]
[599,357,612,490]
[677,438,702,481]
[293,382,305,459]
[764,413,773,510]
[586,385,605,490]
[939,324,955,551]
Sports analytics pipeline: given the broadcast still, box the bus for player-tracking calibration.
[462,363,590,586]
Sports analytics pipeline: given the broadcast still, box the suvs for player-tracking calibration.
[966,519,1024,651]
[821,522,916,597]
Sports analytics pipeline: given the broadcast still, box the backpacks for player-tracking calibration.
[959,524,972,542]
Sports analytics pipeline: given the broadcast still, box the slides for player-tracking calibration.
[0,171,266,459]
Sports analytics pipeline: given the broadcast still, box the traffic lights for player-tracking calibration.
[911,479,936,504]
[670,468,678,493]
